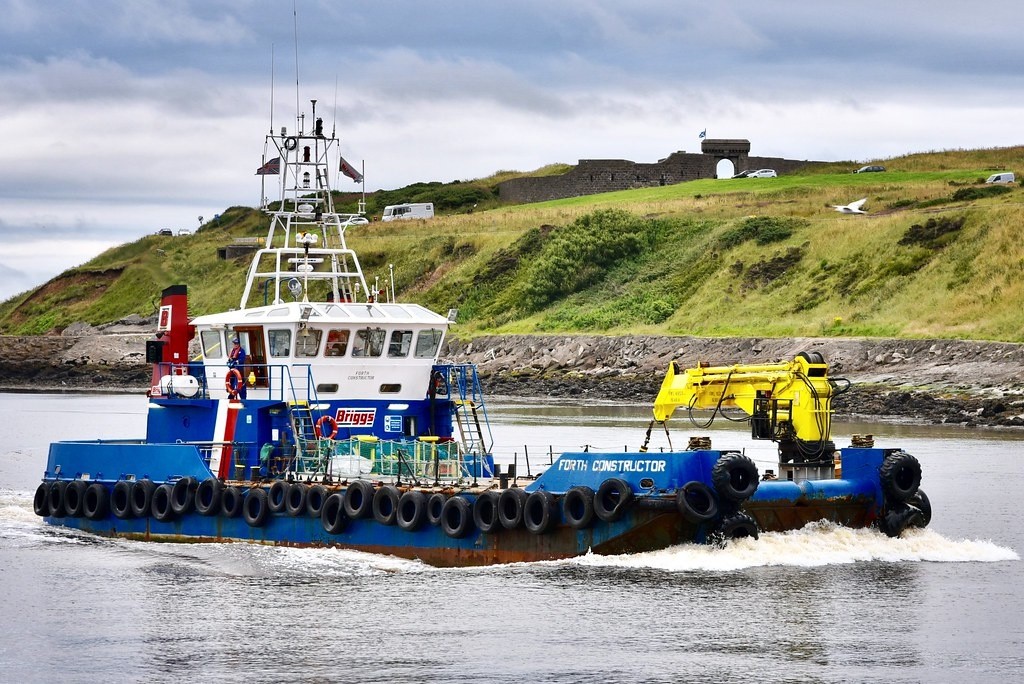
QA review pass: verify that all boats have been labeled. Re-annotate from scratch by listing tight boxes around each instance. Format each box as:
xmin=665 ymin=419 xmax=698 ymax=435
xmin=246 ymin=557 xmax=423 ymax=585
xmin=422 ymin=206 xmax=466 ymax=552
xmin=33 ymin=2 xmax=931 ymax=566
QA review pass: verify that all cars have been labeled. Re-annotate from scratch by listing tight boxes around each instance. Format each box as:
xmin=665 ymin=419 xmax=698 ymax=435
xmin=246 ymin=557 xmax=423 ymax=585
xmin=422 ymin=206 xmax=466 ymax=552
xmin=177 ymin=229 xmax=191 ymax=236
xmin=855 ymin=165 xmax=887 ymax=174
xmin=731 ymin=168 xmax=778 ymax=179
xmin=158 ymin=228 xmax=173 ymax=235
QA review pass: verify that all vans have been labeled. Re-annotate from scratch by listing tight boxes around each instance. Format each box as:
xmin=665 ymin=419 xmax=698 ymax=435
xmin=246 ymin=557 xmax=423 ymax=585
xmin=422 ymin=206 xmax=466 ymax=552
xmin=985 ymin=173 xmax=1015 ymax=185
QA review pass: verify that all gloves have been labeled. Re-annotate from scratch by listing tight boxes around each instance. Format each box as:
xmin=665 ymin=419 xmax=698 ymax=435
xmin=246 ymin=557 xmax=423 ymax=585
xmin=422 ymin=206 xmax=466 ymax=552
xmin=228 ymin=362 xmax=231 ymax=368
xmin=230 ymin=361 xmax=237 ymax=368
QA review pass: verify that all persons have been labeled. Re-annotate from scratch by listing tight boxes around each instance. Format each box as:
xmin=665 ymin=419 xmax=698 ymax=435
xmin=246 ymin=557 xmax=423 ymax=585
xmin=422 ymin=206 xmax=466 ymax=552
xmin=226 ymin=339 xmax=246 ymax=400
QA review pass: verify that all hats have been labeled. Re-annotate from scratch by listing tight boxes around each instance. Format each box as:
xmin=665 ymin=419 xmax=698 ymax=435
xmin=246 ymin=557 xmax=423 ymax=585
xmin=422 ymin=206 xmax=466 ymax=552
xmin=232 ymin=339 xmax=240 ymax=343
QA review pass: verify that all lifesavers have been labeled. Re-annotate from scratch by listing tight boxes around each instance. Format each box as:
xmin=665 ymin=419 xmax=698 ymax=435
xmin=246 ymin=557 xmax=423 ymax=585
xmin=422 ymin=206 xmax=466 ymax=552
xmin=676 ymin=480 xmax=719 ymax=524
xmin=882 ymin=488 xmax=932 ymax=539
xmin=343 ymin=480 xmax=473 ymax=538
xmin=33 ymin=480 xmax=110 ymax=521
xmin=713 ymin=510 xmax=760 ymax=550
xmin=225 ymin=367 xmax=243 ymax=396
xmin=472 ymin=487 xmax=560 ymax=536
xmin=110 ymin=476 xmax=268 ymax=527
xmin=563 ymin=476 xmax=635 ymax=531
xmin=711 ymin=451 xmax=760 ymax=503
xmin=267 ymin=480 xmax=350 ymax=535
xmin=315 ymin=415 xmax=338 ymax=439
xmin=879 ymin=450 xmax=923 ymax=502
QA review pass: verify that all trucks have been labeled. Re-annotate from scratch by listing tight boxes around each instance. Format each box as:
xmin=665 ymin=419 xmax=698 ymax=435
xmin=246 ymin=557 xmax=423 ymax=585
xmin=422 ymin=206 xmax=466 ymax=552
xmin=382 ymin=202 xmax=434 ymax=223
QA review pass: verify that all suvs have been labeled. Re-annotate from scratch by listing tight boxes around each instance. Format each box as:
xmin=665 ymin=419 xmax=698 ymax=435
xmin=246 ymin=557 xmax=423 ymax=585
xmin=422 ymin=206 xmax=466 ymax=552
xmin=342 ymin=217 xmax=369 ymax=226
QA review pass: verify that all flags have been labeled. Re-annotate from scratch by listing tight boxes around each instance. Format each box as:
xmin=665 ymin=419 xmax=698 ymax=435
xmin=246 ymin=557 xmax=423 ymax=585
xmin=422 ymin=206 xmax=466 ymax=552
xmin=699 ymin=131 xmax=705 ymax=138
xmin=339 ymin=156 xmax=363 ymax=183
xmin=256 ymin=156 xmax=280 ymax=175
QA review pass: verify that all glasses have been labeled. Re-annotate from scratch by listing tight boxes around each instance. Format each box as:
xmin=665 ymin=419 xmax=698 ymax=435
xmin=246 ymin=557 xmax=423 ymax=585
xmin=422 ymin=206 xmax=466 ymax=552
xmin=234 ymin=342 xmax=239 ymax=345
